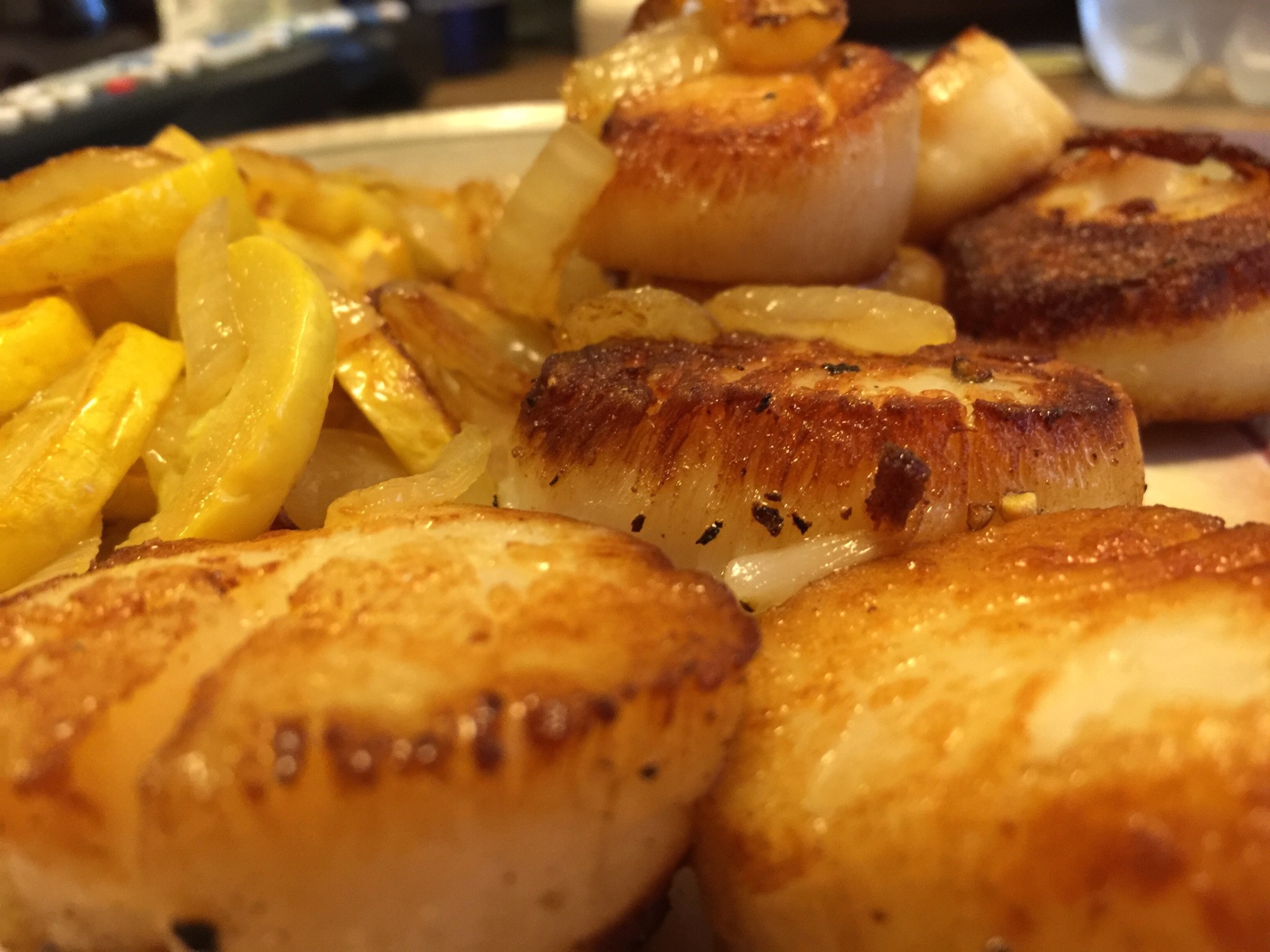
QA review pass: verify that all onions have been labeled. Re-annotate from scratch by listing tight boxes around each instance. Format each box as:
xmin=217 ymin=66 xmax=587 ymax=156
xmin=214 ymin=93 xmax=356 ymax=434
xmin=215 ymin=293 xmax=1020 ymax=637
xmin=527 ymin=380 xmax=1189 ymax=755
xmin=313 ymin=20 xmax=955 ymax=602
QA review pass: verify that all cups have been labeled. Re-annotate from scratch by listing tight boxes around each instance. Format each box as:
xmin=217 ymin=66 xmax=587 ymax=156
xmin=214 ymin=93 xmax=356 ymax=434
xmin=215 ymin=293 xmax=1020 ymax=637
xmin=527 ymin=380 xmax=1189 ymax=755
xmin=1225 ymin=0 xmax=1270 ymax=108
xmin=1079 ymin=0 xmax=1239 ymax=103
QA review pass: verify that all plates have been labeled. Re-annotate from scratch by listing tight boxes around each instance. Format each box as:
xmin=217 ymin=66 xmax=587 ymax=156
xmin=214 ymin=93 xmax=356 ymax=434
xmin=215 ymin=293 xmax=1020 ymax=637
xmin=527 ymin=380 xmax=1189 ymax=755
xmin=228 ymin=99 xmax=1269 ymax=531
